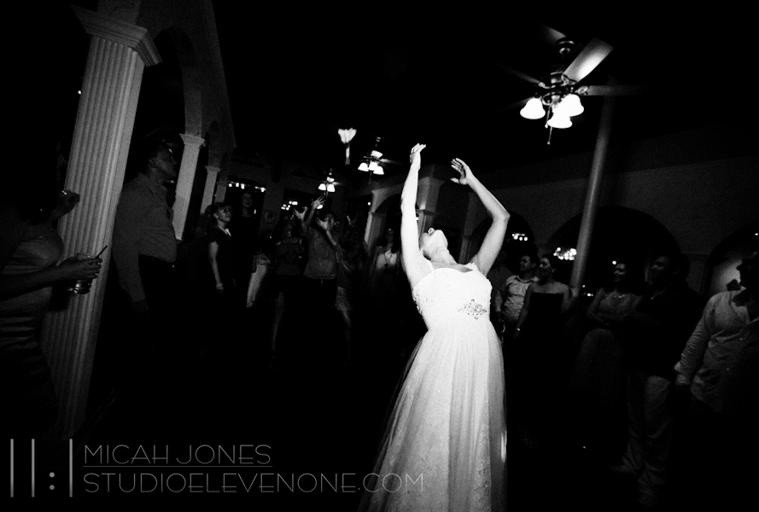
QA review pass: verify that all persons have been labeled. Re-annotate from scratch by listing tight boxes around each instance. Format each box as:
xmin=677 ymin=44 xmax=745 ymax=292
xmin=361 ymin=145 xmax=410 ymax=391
xmin=0 ymin=176 xmax=101 ymax=449
xmin=82 ymin=138 xmax=183 ymax=442
xmin=360 ymin=141 xmax=513 ymax=511
xmin=196 ymin=188 xmax=401 ymax=361
xmin=489 ymin=241 xmax=759 ymax=511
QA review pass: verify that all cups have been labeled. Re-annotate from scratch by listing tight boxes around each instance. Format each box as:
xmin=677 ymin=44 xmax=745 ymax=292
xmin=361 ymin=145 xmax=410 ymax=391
xmin=73 ymin=252 xmax=93 ymax=294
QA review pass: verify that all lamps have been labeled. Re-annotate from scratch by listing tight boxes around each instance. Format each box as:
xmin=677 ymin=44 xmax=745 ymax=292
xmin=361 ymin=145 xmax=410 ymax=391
xmin=318 ymin=176 xmax=337 ymax=195
xmin=524 ymin=92 xmax=584 ymax=131
xmin=357 ymin=161 xmax=387 ymax=176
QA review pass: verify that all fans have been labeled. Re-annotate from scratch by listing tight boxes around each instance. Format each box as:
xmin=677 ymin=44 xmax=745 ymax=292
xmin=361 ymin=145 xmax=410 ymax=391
xmin=495 ymin=34 xmax=624 ymax=135
xmin=353 ymin=150 xmax=396 ymax=180
